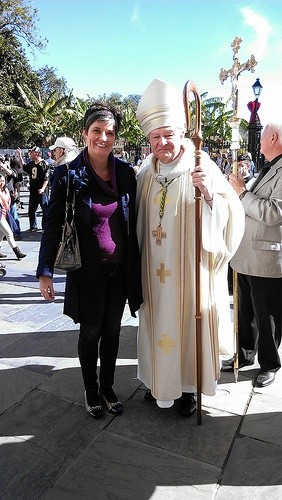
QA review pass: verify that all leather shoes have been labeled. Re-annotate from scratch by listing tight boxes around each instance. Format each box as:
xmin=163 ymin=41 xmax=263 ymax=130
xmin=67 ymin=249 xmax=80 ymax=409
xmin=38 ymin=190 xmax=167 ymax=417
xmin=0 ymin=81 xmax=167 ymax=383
xmin=221 ymin=360 xmax=246 ymax=372
xmin=254 ymin=369 xmax=275 ymax=387
xmin=145 ymin=388 xmax=157 ymax=403
xmin=177 ymin=400 xmax=197 ymax=418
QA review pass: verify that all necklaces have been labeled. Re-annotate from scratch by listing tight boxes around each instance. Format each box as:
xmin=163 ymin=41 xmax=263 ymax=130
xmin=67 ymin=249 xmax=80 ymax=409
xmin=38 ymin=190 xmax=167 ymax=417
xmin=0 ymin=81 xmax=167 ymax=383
xmin=152 ymin=187 xmax=167 ymax=245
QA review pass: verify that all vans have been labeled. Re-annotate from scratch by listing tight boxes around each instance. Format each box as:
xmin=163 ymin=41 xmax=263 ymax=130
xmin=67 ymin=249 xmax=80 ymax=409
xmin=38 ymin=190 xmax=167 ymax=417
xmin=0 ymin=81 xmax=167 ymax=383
xmin=112 ymin=145 xmax=125 ymax=159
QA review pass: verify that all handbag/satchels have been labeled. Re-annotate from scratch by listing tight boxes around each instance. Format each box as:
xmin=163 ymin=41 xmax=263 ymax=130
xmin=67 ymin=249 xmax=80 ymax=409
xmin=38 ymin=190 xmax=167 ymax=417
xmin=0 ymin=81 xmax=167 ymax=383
xmin=6 ymin=209 xmax=19 ymax=233
xmin=54 ymin=162 xmax=82 ymax=272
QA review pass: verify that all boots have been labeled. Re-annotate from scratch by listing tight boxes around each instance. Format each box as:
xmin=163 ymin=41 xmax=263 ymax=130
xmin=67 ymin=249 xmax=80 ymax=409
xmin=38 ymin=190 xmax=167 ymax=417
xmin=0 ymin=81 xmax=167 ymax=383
xmin=0 ymin=246 xmax=7 ymax=258
xmin=13 ymin=246 xmax=26 ymax=260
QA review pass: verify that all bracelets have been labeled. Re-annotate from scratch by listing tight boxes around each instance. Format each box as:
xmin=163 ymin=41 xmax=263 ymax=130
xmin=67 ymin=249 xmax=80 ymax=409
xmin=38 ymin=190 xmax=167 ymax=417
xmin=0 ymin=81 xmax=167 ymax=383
xmin=204 ymin=196 xmax=213 ymax=201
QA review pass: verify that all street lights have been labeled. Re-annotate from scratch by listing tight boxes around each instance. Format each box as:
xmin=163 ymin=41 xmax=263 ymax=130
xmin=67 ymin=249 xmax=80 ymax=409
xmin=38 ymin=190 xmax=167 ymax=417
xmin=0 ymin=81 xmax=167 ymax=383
xmin=247 ymin=78 xmax=264 ymax=172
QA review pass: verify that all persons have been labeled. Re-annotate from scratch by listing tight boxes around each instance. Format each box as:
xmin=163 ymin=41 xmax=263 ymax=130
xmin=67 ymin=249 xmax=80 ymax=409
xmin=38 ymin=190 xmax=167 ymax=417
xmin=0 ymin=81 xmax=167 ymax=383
xmin=0 ymin=152 xmax=27 ymax=260
xmin=49 ymin=137 xmax=80 ymax=187
xmin=22 ymin=149 xmax=56 ymax=166
xmin=16 ymin=146 xmax=50 ymax=233
xmin=119 ymin=148 xmax=256 ymax=183
xmin=137 ymin=79 xmax=246 ymax=414
xmin=36 ymin=102 xmax=143 ymax=418
xmin=222 ymin=120 xmax=282 ymax=387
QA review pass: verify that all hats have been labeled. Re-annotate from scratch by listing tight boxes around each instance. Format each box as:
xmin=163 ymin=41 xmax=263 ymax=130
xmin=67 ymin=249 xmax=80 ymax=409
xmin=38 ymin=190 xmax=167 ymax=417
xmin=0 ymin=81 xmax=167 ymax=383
xmin=135 ymin=78 xmax=184 ymax=137
xmin=49 ymin=137 xmax=76 ymax=151
xmin=28 ymin=146 xmax=40 ymax=153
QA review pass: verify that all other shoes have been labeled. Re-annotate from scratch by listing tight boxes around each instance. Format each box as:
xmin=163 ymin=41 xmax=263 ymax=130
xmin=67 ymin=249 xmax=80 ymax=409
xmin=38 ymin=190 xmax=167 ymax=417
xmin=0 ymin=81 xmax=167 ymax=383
xmin=100 ymin=387 xmax=123 ymax=416
xmin=14 ymin=232 xmax=21 ymax=241
xmin=26 ymin=227 xmax=38 ymax=233
xmin=83 ymin=390 xmax=104 ymax=419
